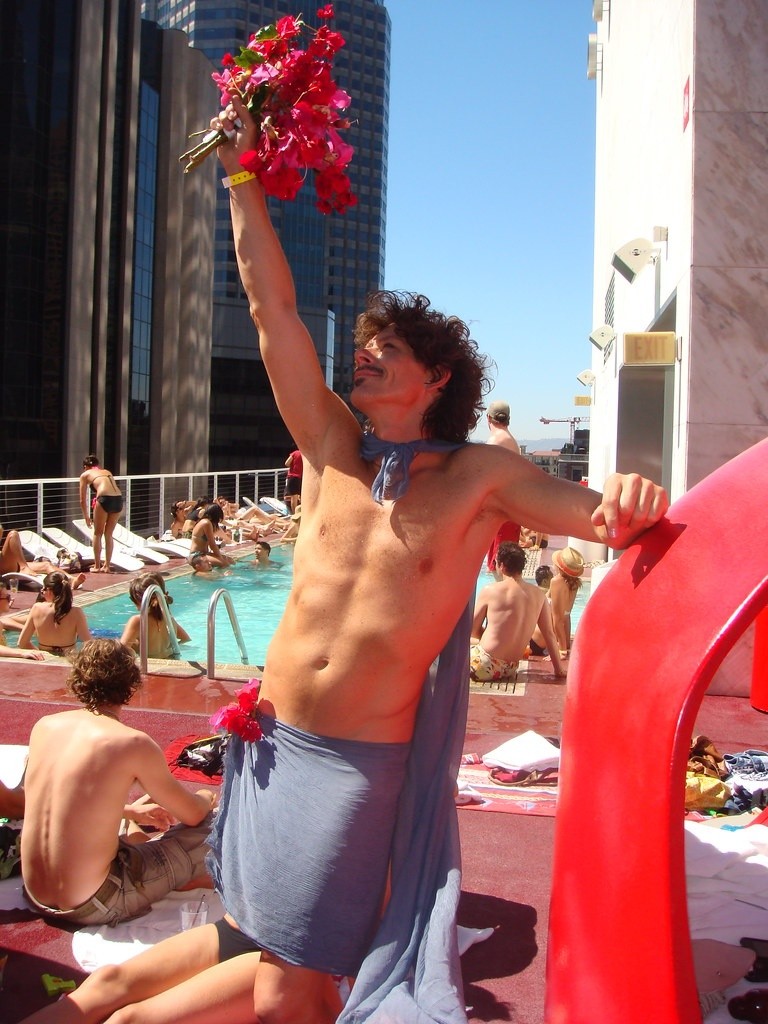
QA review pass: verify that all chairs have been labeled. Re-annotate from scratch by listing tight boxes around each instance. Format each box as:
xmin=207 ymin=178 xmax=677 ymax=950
xmin=243 ymin=496 xmax=270 ymax=517
xmin=17 ymin=530 xmax=62 ymax=567
xmin=160 ymin=530 xmax=193 ymax=550
xmin=72 ymin=518 xmax=170 ymax=564
xmin=112 ymin=522 xmax=192 ymax=559
xmin=42 ymin=527 xmax=145 ymax=572
xmin=260 ymin=497 xmax=289 ymax=516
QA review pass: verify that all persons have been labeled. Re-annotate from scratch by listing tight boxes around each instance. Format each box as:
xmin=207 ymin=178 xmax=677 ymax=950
xmin=118 ymin=578 xmax=177 ymax=660
xmin=0 ymin=761 xmax=30 ymax=883
xmin=17 ymin=571 xmax=94 ymax=656
xmin=0 ymin=582 xmax=46 ymax=660
xmin=78 ymin=455 xmax=122 ymax=574
xmin=19 ymin=637 xmax=219 ymax=926
xmin=168 ymin=443 xmax=302 ymax=577
xmin=466 ymin=403 xmax=582 ymax=679
xmin=138 ymin=571 xmax=173 ymax=613
xmin=205 ymin=104 xmax=670 ymax=1024
xmin=0 ymin=525 xmax=85 ymax=590
xmin=15 ymin=863 xmax=399 ymax=1024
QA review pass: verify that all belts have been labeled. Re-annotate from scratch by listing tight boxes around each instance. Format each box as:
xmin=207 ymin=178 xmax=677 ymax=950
xmin=22 ymin=859 xmax=122 ymax=921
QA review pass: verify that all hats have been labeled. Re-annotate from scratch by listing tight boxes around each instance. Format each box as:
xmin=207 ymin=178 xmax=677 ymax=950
xmin=489 ymin=400 xmax=510 ymax=421
xmin=551 ymin=547 xmax=585 ymax=578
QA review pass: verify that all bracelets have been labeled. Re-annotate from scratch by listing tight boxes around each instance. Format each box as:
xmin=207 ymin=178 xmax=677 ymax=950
xmin=221 ymin=170 xmax=256 ymax=188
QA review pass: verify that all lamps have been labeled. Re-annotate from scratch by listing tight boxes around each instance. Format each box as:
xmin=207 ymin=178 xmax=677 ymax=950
xmin=611 ymin=237 xmax=660 ymax=283
xmin=588 ymin=324 xmax=617 ymax=351
xmin=577 ymin=369 xmax=597 ymax=387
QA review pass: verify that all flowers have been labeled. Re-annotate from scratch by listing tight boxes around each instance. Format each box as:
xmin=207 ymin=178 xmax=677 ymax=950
xmin=210 ymin=677 xmax=264 ymax=745
xmin=177 ymin=4 xmax=363 ymax=219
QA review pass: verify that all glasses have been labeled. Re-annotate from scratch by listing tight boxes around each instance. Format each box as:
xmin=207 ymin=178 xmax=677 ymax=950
xmin=41 ymin=587 xmax=48 ymax=595
xmin=0 ymin=595 xmax=11 ymax=602
